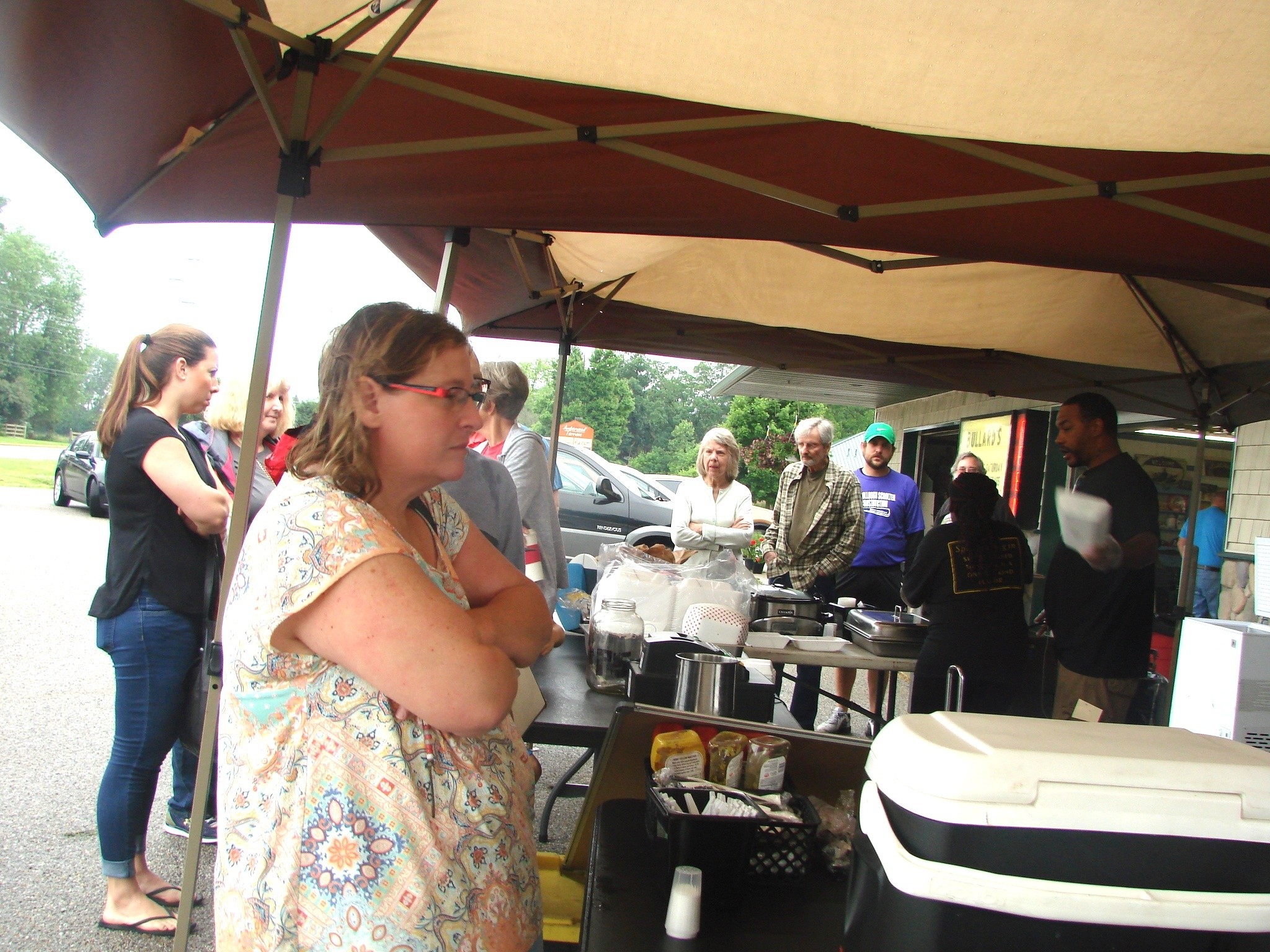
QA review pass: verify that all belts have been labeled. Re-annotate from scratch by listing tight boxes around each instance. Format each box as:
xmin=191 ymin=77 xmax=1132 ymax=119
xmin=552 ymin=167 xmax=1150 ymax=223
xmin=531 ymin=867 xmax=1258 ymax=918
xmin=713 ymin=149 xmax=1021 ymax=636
xmin=1198 ymin=566 xmax=1220 ymax=572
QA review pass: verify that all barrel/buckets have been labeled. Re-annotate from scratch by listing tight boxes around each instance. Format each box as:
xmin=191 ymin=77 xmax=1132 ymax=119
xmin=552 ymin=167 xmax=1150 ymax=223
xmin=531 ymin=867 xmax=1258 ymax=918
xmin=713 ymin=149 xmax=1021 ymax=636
xmin=673 ymin=652 xmax=741 ymax=718
xmin=749 ymin=616 xmax=824 ymax=636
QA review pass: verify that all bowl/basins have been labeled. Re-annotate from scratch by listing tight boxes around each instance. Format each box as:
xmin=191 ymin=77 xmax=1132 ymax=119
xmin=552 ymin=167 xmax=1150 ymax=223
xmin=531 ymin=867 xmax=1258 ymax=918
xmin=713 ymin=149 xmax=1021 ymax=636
xmin=786 ymin=635 xmax=852 ymax=652
xmin=745 ymin=632 xmax=791 ymax=649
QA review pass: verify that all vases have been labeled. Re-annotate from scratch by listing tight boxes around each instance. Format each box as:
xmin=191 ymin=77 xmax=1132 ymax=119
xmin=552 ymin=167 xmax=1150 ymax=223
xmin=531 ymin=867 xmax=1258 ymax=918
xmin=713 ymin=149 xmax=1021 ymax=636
xmin=743 ymin=556 xmax=765 ymax=574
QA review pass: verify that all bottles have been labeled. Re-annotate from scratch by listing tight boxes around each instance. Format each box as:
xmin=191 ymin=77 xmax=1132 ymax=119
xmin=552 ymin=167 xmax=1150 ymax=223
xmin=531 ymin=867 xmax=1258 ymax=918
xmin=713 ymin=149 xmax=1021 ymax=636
xmin=584 ymin=598 xmax=645 ymax=696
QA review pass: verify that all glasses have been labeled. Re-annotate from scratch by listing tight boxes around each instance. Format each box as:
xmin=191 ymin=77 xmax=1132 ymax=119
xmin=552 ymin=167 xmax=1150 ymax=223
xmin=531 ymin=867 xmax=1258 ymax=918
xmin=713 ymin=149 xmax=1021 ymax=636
xmin=383 ymin=382 xmax=487 ymax=411
xmin=474 ymin=377 xmax=492 ymax=391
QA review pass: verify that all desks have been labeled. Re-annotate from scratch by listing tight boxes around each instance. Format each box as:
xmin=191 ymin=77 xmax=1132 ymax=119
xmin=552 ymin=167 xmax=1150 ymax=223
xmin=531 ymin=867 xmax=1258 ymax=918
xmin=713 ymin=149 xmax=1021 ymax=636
xmin=521 ymin=628 xmax=805 ymax=845
xmin=737 ymin=631 xmax=920 ymax=736
xmin=581 ymin=796 xmax=843 ymax=952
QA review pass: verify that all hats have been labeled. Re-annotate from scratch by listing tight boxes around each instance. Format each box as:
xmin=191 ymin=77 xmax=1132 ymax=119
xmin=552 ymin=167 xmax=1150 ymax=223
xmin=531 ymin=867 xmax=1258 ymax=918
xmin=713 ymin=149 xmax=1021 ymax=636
xmin=863 ymin=422 xmax=896 ymax=445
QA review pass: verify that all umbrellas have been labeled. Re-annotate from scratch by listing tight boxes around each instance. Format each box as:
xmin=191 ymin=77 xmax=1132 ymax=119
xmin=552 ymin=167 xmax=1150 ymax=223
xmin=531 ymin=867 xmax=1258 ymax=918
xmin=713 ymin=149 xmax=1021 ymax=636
xmin=0 ymin=0 xmax=1270 ymax=952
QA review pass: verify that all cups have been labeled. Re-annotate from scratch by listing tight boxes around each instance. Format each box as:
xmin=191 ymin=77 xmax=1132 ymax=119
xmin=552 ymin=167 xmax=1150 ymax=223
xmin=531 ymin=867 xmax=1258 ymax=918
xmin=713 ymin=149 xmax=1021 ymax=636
xmin=664 ymin=866 xmax=702 ymax=940
xmin=826 ymin=623 xmax=838 ymax=637
xmin=822 ymin=624 xmax=834 ymax=637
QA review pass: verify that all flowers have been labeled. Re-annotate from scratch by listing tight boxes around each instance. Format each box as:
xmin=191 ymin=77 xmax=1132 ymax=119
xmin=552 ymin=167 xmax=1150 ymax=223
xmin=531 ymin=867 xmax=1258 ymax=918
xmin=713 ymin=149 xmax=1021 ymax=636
xmin=741 ymin=532 xmax=767 ymax=564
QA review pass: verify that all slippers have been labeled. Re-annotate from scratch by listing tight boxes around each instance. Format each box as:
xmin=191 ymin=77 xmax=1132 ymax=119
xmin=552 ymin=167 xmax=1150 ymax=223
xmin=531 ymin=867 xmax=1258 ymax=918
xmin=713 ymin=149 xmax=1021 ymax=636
xmin=99 ymin=908 xmax=197 ymax=936
xmin=147 ymin=883 xmax=203 ymax=905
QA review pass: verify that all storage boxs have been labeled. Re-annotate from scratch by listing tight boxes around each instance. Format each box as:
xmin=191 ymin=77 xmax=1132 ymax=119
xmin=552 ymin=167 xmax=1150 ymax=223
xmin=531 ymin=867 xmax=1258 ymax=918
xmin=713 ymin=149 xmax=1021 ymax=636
xmin=843 ymin=710 xmax=1270 ymax=952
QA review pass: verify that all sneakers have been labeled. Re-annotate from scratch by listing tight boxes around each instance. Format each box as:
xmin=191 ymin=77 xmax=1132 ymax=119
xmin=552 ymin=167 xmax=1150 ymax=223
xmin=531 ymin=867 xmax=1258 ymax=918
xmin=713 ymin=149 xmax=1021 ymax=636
xmin=869 ymin=718 xmax=883 ymax=738
xmin=817 ymin=707 xmax=851 ymax=732
xmin=164 ymin=806 xmax=218 ymax=843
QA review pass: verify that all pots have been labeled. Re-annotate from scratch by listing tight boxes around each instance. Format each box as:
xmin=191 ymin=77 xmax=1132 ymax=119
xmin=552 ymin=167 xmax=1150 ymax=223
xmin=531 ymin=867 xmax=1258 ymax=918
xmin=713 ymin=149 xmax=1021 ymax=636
xmin=749 ymin=583 xmax=823 ymax=621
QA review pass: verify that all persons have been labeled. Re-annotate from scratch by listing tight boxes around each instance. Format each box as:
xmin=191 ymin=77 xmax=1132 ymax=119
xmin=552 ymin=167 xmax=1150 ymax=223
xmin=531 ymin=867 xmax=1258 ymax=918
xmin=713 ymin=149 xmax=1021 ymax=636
xmin=527 ymin=620 xmax=565 ymax=785
xmin=759 ymin=416 xmax=866 ymax=731
xmin=816 ymin=423 xmax=926 ymax=737
xmin=1031 ymin=392 xmax=1164 ymax=724
xmin=1176 ymin=487 xmax=1227 ymax=619
xmin=213 ymin=301 xmax=553 ymax=952
xmin=671 ymin=427 xmax=754 ymax=566
xmin=900 ymin=472 xmax=1034 ymax=715
xmin=439 ymin=447 xmax=525 ymax=577
xmin=89 ymin=323 xmax=231 ymax=935
xmin=465 ymin=361 xmax=569 ymax=617
xmin=934 ymin=452 xmax=1017 ymax=527
xmin=166 ymin=353 xmax=296 ymax=842
xmin=518 ymin=423 xmax=563 ymax=514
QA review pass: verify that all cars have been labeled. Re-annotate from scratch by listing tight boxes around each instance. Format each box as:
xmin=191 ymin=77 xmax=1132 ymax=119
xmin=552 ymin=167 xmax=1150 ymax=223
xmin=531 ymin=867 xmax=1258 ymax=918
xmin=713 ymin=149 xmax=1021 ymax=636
xmin=541 ymin=438 xmax=681 ymax=568
xmin=53 ymin=430 xmax=113 ymax=518
xmin=608 ymin=464 xmax=775 ymax=541
